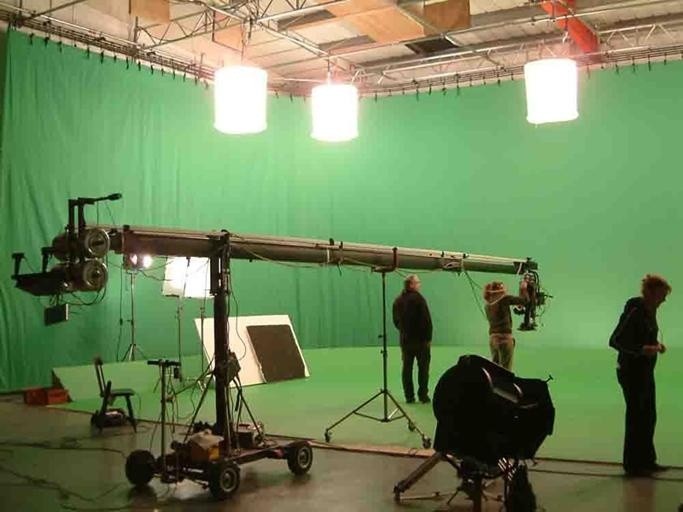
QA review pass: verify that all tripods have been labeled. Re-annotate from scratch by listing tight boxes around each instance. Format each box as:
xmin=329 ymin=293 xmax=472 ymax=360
xmin=324 ymin=273 xmax=431 ymax=448
xmin=122 ymin=274 xmax=148 ymax=362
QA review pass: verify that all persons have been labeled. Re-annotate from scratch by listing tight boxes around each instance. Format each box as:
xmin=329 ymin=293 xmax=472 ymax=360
xmin=483 ymin=279 xmax=529 ymax=374
xmin=609 ymin=276 xmax=672 ymax=476
xmin=392 ymin=275 xmax=433 ymax=404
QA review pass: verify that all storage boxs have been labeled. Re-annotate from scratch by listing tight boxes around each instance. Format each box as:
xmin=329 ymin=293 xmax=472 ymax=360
xmin=24 ymin=389 xmax=68 ymax=405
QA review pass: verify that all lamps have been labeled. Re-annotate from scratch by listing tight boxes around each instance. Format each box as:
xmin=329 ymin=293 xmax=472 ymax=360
xmin=311 ymin=57 xmax=358 ymax=142
xmin=523 ymin=15 xmax=579 ymax=124
xmin=213 ymin=20 xmax=267 ymax=135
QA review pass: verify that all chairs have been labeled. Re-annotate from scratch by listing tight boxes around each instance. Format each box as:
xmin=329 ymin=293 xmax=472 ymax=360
xmin=94 ymin=355 xmax=138 ymax=434
